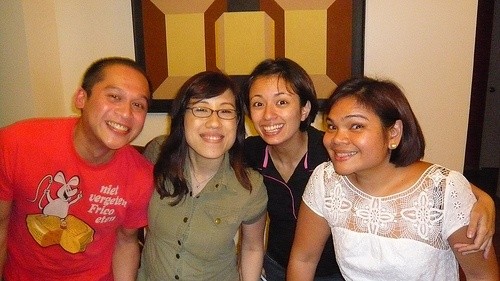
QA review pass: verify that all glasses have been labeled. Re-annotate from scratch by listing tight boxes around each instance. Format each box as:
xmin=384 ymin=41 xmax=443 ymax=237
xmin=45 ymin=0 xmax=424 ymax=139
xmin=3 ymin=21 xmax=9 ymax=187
xmin=185 ymin=105 xmax=241 ymax=121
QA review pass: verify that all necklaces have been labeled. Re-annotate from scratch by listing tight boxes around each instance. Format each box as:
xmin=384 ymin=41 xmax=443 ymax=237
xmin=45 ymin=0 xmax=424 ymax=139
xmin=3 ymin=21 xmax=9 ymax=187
xmin=189 ymin=165 xmax=215 ymax=190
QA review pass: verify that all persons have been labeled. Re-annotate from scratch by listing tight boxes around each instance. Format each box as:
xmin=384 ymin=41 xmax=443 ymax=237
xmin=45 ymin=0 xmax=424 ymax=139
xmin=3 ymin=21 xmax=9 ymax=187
xmin=0 ymin=56 xmax=156 ymax=281
xmin=237 ymin=57 xmax=495 ymax=281
xmin=133 ymin=70 xmax=269 ymax=281
xmin=287 ymin=77 xmax=500 ymax=281
